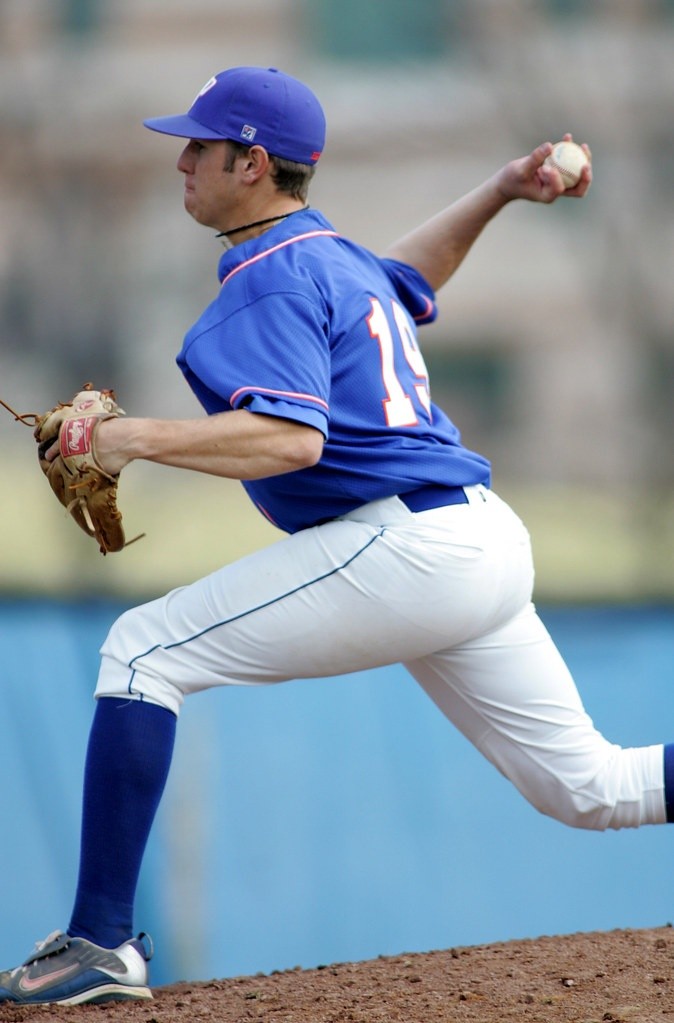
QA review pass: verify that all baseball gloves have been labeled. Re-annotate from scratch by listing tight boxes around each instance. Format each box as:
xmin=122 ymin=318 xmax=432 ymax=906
xmin=33 ymin=380 xmax=129 ymax=557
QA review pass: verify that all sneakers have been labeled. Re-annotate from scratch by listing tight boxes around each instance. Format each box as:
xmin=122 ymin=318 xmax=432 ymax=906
xmin=0 ymin=930 xmax=153 ymax=1006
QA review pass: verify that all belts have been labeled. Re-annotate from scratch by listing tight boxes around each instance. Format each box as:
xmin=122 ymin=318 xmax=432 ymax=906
xmin=397 ymin=485 xmax=468 ymax=514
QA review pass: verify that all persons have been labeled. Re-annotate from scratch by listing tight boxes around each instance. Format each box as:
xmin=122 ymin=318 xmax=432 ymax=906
xmin=0 ymin=65 xmax=674 ymax=1006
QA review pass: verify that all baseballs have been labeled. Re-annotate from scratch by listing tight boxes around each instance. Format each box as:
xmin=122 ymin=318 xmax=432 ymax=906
xmin=534 ymin=138 xmax=589 ymax=192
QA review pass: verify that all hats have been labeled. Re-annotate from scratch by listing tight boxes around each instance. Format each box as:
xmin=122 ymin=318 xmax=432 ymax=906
xmin=143 ymin=67 xmax=326 ymax=165
